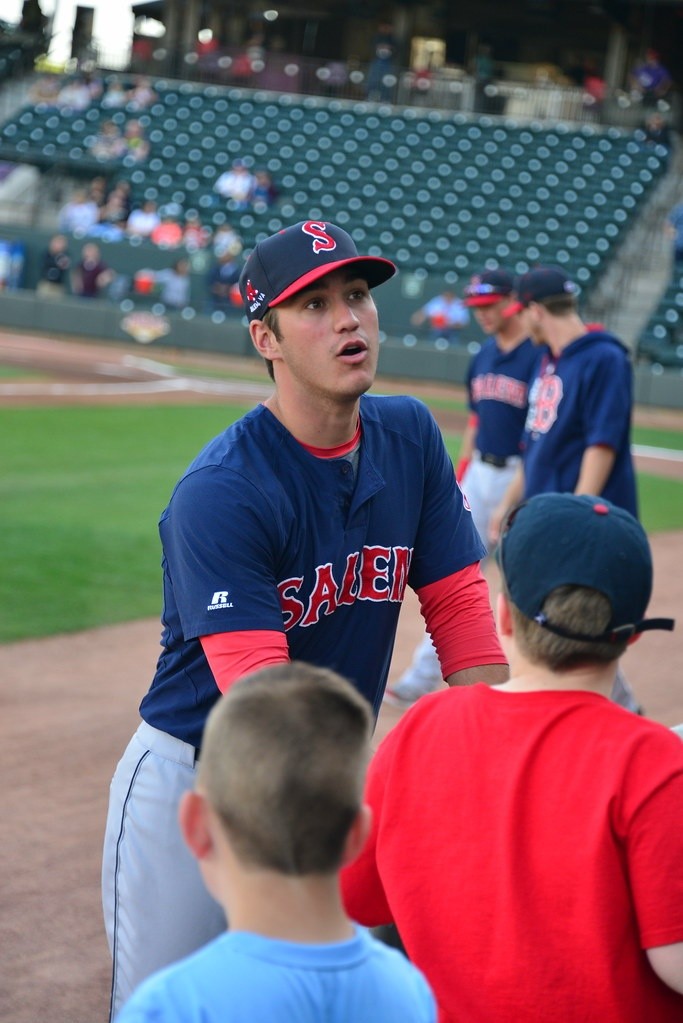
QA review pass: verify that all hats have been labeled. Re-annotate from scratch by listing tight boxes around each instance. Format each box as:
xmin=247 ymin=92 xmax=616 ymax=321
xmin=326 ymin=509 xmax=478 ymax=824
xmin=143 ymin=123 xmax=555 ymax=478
xmin=500 ymin=268 xmax=575 ymax=317
xmin=495 ymin=492 xmax=674 ymax=641
xmin=463 ymin=268 xmax=513 ymax=305
xmin=237 ymin=220 xmax=396 ymax=323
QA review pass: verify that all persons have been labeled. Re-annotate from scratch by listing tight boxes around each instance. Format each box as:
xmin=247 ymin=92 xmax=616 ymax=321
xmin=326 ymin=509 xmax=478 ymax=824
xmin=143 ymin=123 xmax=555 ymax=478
xmin=0 ymin=176 xmax=249 ymax=319
xmin=132 ymin=26 xmax=674 ymax=148
xmin=338 ymin=493 xmax=683 ymax=1023
xmin=213 ymin=160 xmax=276 ymax=210
xmin=488 ymin=272 xmax=647 ymax=715
xmin=666 ymin=200 xmax=683 ymax=263
xmin=113 ymin=661 xmax=438 ymax=1023
xmin=103 ymin=221 xmax=508 ymax=1023
xmin=30 ymin=70 xmax=157 ymax=113
xmin=383 ymin=269 xmax=548 ymax=706
xmin=90 ymin=120 xmax=150 ymax=164
xmin=409 ymin=285 xmax=469 ymax=345
